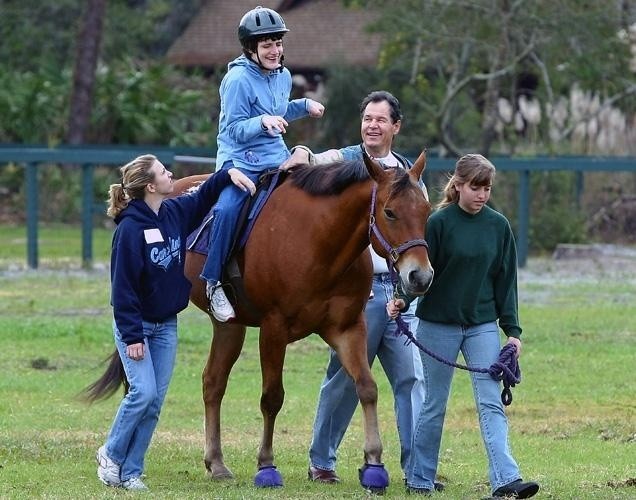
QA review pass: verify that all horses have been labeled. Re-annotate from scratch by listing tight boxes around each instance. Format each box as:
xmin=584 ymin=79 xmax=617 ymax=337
xmin=71 ymin=143 xmax=435 ymax=497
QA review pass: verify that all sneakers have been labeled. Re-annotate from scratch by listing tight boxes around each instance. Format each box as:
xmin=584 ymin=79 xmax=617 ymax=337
xmin=95 ymin=445 xmax=122 ymax=486
xmin=117 ymin=475 xmax=148 ymax=493
xmin=206 ymin=278 xmax=238 ymax=323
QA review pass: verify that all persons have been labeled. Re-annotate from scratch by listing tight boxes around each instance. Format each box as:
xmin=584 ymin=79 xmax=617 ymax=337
xmin=396 ymin=151 xmax=540 ymax=500
xmin=276 ymin=87 xmax=448 ymax=494
xmin=93 ymin=153 xmax=259 ymax=492
xmin=188 ymin=5 xmax=327 ymax=326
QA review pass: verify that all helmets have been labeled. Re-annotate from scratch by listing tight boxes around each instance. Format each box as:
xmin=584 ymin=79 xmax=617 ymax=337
xmin=237 ymin=4 xmax=290 ymax=42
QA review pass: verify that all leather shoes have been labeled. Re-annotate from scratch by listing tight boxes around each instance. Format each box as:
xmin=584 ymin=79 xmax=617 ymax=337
xmin=491 ymin=480 xmax=540 ymax=500
xmin=308 ymin=463 xmax=342 ymax=486
xmin=401 ymin=473 xmax=446 ymax=497
